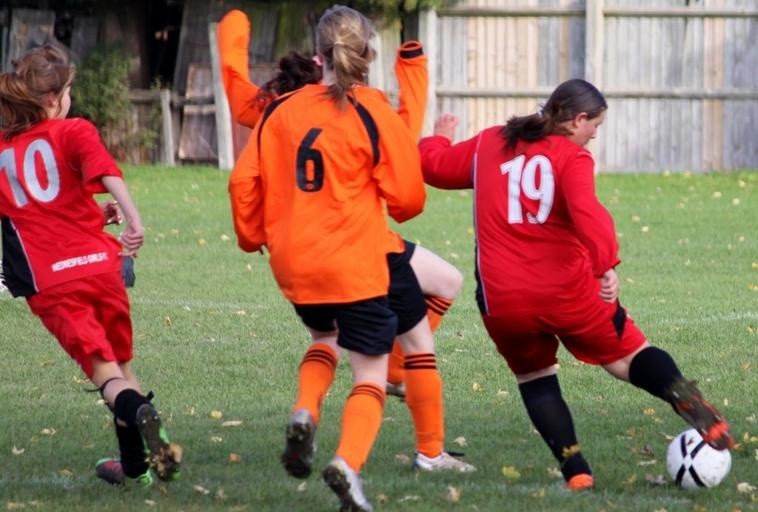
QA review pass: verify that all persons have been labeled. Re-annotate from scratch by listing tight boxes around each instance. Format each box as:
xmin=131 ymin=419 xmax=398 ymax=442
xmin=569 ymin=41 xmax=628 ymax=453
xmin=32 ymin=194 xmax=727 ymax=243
xmin=225 ymin=4 xmax=429 ymax=512
xmin=216 ymin=9 xmax=480 ymax=478
xmin=417 ymin=77 xmax=738 ymax=494
xmin=0 ymin=39 xmax=185 ymax=492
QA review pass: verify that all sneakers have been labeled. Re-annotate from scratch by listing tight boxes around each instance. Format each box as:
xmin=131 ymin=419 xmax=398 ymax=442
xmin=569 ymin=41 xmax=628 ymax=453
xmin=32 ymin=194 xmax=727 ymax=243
xmin=322 ymin=457 xmax=374 ymax=512
xmin=567 ymin=471 xmax=593 ymax=491
xmin=386 ymin=380 xmax=403 ymax=399
xmin=667 ymin=377 xmax=733 ymax=451
xmin=95 ymin=458 xmax=154 ymax=488
xmin=129 ymin=397 xmax=183 ymax=483
xmin=280 ymin=411 xmax=314 ymax=478
xmin=415 ymin=452 xmax=477 ymax=473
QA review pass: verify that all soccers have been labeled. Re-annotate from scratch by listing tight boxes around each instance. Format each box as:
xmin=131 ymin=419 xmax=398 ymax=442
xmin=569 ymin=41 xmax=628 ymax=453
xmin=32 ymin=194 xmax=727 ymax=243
xmin=667 ymin=428 xmax=731 ymax=490
